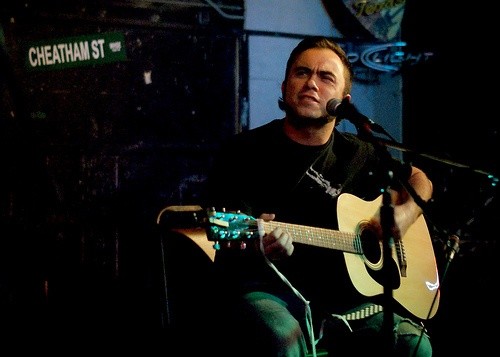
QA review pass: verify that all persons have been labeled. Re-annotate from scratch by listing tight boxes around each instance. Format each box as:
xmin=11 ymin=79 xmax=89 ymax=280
xmin=179 ymin=35 xmax=436 ymax=357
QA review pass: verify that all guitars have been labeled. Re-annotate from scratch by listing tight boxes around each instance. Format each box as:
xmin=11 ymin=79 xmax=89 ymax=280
xmin=156 ymin=182 xmax=440 ymax=320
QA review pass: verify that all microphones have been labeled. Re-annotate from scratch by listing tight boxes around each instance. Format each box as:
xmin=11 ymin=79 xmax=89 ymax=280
xmin=444 ymin=235 xmax=461 ymax=262
xmin=326 ymin=98 xmax=385 ymax=135
